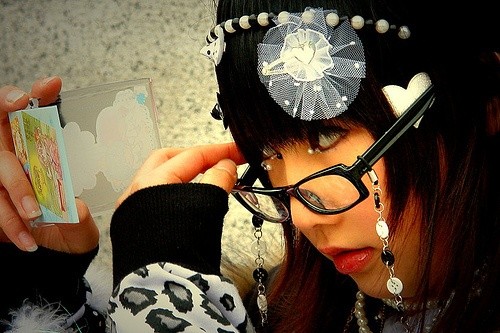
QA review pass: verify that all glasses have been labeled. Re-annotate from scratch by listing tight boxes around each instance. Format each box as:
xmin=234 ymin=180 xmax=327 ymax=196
xmin=229 ymin=81 xmax=449 ymax=223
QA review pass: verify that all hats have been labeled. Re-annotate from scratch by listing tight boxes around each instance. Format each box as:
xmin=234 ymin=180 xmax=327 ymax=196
xmin=211 ymin=0 xmax=500 ymax=137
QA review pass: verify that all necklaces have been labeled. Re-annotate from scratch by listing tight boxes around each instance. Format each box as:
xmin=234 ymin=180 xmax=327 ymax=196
xmin=353 ymin=290 xmax=374 ymax=333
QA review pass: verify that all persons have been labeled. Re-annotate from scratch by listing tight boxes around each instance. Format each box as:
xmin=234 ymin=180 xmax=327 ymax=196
xmin=0 ymin=0 xmax=500 ymax=333
